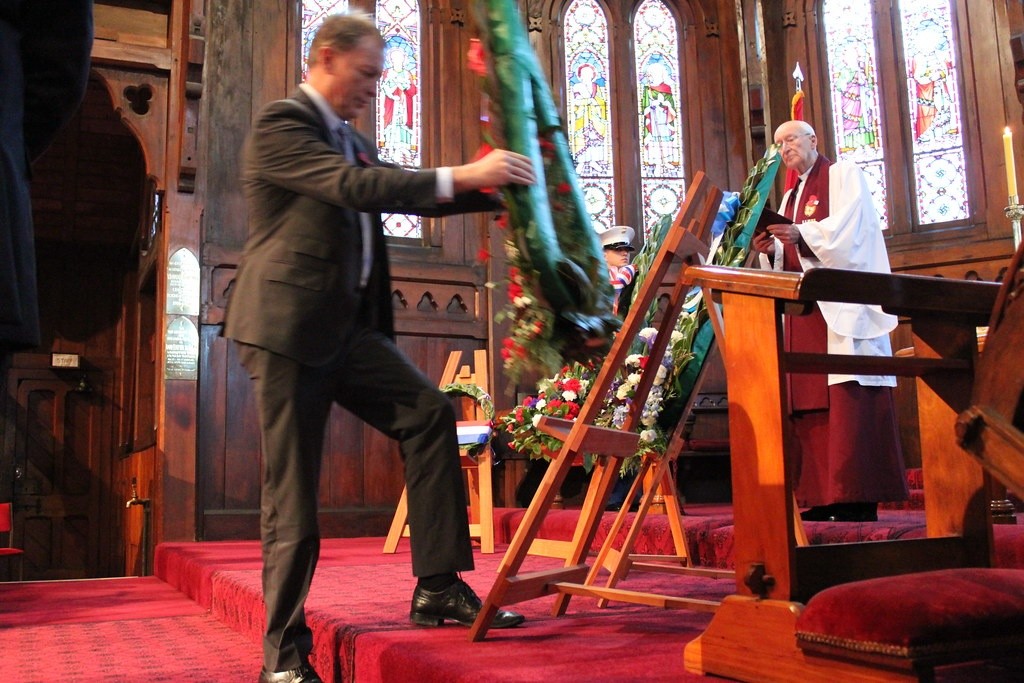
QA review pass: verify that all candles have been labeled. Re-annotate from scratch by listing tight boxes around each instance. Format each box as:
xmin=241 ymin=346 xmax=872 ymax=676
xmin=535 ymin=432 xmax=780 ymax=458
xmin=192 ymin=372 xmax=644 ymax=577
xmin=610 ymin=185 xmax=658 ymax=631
xmin=1002 ymin=127 xmax=1020 ymax=205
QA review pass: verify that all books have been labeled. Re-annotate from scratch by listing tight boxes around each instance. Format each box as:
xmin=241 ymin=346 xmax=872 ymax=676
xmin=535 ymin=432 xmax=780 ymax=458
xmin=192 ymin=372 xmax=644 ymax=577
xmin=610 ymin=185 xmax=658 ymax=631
xmin=754 ymin=207 xmax=795 ymax=238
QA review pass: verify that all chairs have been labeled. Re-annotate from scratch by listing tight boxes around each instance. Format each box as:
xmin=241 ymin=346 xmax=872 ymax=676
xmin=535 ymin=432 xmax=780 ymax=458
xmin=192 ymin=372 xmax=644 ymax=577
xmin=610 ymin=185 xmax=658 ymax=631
xmin=0 ymin=503 xmax=24 ymax=581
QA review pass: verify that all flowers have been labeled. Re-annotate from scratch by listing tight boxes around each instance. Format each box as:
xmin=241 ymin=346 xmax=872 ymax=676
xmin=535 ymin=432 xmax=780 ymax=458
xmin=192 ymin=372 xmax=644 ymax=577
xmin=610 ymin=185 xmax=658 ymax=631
xmin=489 ymin=325 xmax=685 ymax=464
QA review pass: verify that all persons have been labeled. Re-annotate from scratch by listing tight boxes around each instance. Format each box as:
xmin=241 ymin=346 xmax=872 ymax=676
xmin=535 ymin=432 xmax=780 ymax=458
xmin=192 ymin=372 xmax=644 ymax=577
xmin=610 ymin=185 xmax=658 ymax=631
xmin=217 ymin=15 xmax=537 ymax=683
xmin=597 ymin=226 xmax=640 ymax=325
xmin=0 ymin=0 xmax=96 ymax=430
xmin=749 ymin=119 xmax=909 ymax=523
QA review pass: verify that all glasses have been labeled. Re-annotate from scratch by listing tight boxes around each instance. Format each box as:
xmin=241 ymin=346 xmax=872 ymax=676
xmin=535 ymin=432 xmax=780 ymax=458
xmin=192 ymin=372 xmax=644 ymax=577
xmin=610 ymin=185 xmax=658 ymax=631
xmin=775 ymin=133 xmax=810 ymax=149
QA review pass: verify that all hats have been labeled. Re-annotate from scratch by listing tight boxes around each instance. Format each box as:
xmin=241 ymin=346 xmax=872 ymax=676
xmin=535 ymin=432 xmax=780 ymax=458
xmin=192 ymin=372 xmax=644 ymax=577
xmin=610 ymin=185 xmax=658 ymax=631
xmin=599 ymin=226 xmax=636 ymax=251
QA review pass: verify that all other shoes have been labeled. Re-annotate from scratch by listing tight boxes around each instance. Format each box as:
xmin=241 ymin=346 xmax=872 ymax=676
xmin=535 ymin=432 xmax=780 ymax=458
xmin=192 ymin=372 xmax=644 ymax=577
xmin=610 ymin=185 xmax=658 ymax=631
xmin=801 ymin=500 xmax=879 ymax=522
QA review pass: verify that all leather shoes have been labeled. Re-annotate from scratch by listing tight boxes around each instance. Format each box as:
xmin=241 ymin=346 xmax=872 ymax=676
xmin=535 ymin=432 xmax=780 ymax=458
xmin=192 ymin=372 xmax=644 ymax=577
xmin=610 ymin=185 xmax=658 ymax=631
xmin=258 ymin=659 xmax=324 ymax=683
xmin=409 ymin=579 xmax=526 ymax=628
